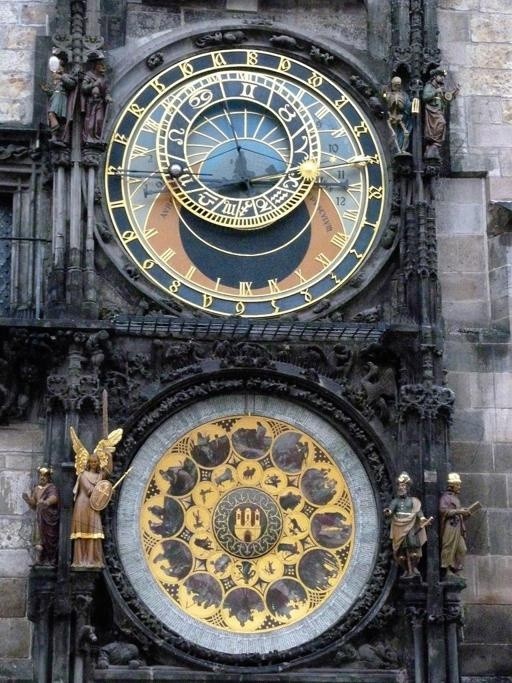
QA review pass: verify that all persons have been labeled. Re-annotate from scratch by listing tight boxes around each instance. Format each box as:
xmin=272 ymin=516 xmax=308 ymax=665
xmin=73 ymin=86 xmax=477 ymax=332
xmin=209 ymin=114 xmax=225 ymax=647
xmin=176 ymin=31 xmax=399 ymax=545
xmin=23 ymin=465 xmax=61 ymax=565
xmin=422 ymin=65 xmax=459 ymax=158
xmin=439 ymin=471 xmax=471 ymax=583
xmin=381 ymin=470 xmax=428 ymax=584
xmin=40 ymin=54 xmax=76 ymax=138
xmin=70 ymin=452 xmax=107 ymax=566
xmin=82 ymin=48 xmax=111 ymax=140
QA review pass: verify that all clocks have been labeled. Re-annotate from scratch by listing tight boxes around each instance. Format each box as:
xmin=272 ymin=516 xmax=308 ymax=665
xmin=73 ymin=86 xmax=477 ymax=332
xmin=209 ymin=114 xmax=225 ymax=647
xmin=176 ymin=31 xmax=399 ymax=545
xmin=105 ymin=47 xmax=402 ymax=336
